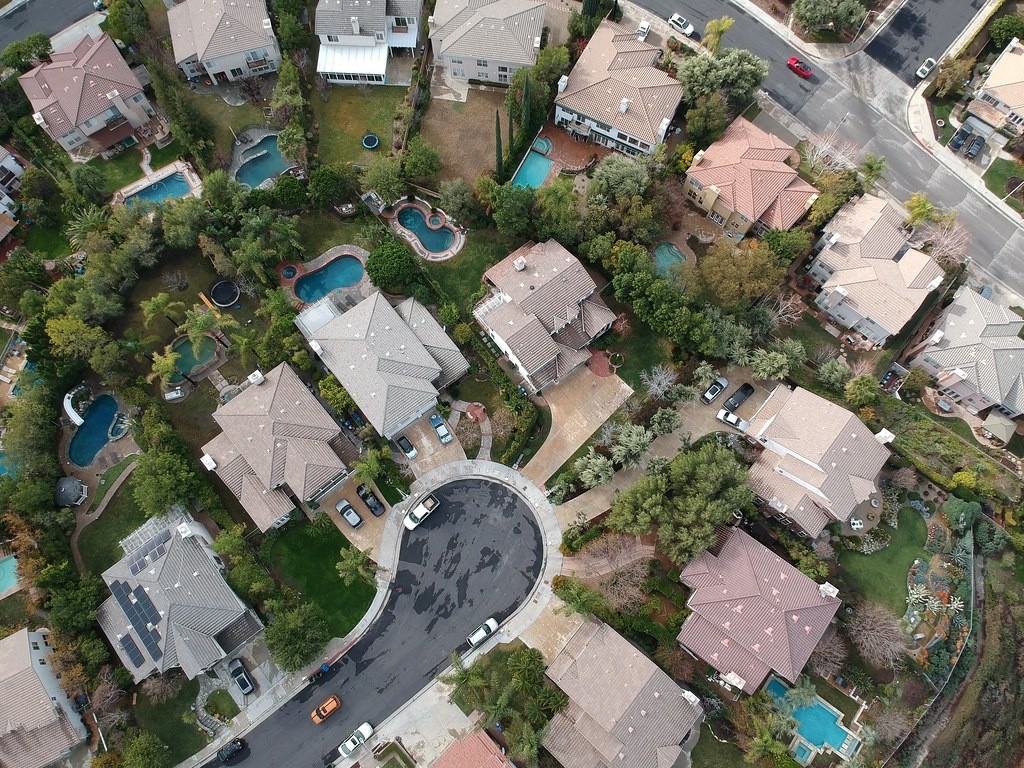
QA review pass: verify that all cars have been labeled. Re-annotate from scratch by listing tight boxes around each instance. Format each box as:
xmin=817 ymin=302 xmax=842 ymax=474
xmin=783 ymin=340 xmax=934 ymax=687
xmin=338 ymin=722 xmax=374 ymax=758
xmin=667 ymin=12 xmax=694 ymax=37
xmin=356 ymin=482 xmax=386 ymax=517
xmin=723 ymin=383 xmax=755 ymax=414
xmin=396 ymin=435 xmax=419 ymax=460
xmin=466 ymin=618 xmax=499 ymax=649
xmin=699 ymin=376 xmax=728 ymax=405
xmin=429 ymin=413 xmax=453 ymax=444
xmin=311 ymin=694 xmax=342 ymax=724
xmin=228 ymin=658 xmax=255 ymax=695
xmin=335 ymin=498 xmax=365 ymax=530
xmin=217 ymin=738 xmax=243 ymax=763
xmin=636 ymin=20 xmax=651 ymax=42
xmin=916 ymin=57 xmax=937 ymax=79
xmin=786 ymin=57 xmax=812 ymax=79
xmin=715 ymin=408 xmax=750 ymax=433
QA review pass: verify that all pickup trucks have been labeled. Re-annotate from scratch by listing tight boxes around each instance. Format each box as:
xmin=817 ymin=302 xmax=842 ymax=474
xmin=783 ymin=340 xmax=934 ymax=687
xmin=402 ymin=493 xmax=440 ymax=531
